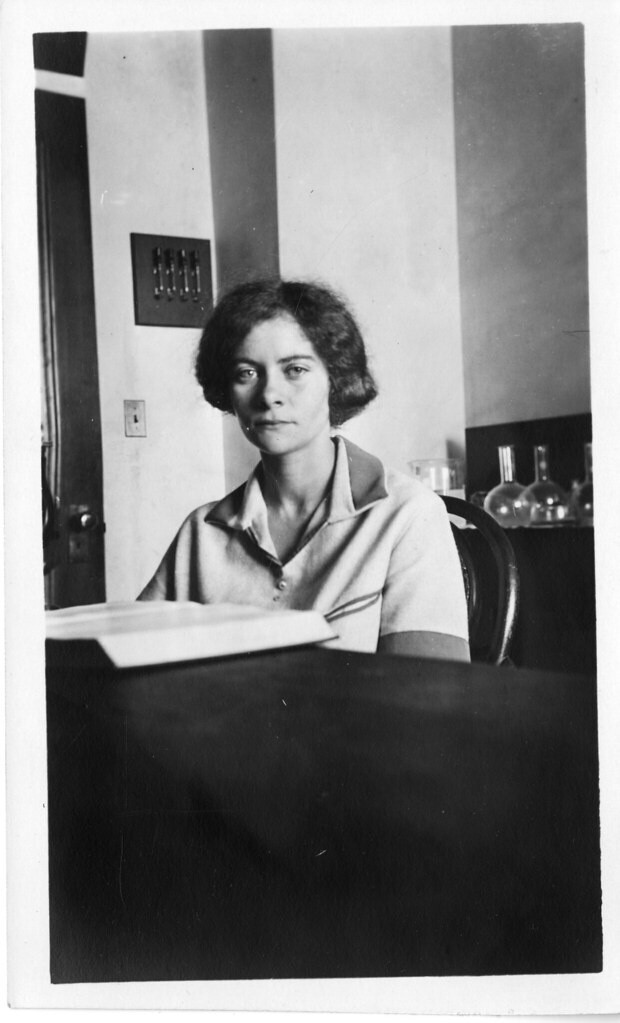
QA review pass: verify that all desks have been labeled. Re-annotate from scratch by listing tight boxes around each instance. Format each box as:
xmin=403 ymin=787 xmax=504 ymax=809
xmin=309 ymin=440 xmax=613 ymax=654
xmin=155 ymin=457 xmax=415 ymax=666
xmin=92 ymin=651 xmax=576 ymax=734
xmin=46 ymin=640 xmax=603 ymax=985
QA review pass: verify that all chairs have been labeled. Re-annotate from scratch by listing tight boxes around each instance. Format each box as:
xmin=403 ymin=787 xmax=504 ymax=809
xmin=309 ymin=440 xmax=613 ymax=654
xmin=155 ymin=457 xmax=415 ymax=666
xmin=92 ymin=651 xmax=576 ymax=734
xmin=441 ymin=496 xmax=520 ymax=668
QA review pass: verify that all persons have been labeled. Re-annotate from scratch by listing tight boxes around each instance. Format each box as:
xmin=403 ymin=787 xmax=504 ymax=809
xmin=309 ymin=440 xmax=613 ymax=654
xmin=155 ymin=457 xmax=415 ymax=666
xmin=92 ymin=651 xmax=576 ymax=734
xmin=132 ymin=279 xmax=471 ymax=662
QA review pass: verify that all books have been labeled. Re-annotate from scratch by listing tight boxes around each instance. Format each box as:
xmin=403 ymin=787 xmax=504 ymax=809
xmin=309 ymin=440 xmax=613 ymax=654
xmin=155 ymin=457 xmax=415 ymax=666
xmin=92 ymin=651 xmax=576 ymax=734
xmin=44 ymin=600 xmax=338 ymax=675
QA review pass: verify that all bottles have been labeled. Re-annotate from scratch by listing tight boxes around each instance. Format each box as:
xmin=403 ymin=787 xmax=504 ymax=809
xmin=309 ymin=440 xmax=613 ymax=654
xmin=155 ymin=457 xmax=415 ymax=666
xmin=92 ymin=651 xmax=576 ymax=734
xmin=483 ymin=443 xmax=533 ymax=527
xmin=514 ymin=444 xmax=569 ymax=527
xmin=571 ymin=441 xmax=594 ymax=527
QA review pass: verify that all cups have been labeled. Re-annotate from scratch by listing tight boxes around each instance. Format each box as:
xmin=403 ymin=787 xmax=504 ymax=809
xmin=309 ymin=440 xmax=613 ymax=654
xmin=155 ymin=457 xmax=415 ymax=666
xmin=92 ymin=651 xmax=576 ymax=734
xmin=409 ymin=458 xmax=465 ymax=500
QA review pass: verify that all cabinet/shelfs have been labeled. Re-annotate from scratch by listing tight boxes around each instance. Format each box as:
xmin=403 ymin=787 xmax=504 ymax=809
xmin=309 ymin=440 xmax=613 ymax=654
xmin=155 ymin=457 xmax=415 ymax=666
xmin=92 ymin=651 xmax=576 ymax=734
xmin=458 ymin=413 xmax=597 ymax=673
